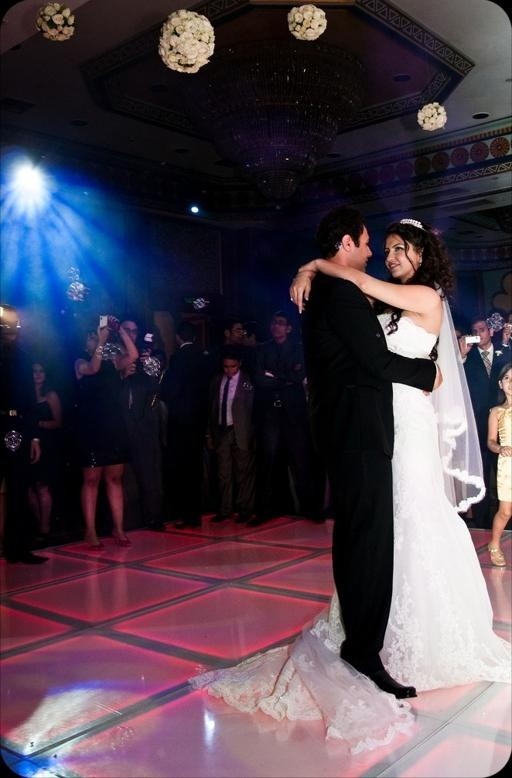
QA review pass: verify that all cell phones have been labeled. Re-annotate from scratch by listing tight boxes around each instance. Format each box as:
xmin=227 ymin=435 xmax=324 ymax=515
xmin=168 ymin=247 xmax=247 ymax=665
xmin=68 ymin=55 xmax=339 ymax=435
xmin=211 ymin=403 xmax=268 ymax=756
xmin=465 ymin=336 xmax=481 ymax=344
xmin=99 ymin=316 xmax=107 ymax=328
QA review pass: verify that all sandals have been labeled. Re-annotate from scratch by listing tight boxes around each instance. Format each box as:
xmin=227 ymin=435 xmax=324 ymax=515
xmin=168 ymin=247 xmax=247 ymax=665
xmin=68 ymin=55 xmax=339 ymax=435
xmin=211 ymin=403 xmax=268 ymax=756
xmin=486 ymin=541 xmax=507 ymax=569
xmin=85 ymin=530 xmax=132 ymax=548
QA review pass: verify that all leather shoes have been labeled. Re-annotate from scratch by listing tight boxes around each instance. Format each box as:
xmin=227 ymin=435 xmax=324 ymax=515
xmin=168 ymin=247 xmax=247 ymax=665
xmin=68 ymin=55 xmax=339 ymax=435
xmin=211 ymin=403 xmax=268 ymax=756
xmin=339 ymin=664 xmax=419 ymax=698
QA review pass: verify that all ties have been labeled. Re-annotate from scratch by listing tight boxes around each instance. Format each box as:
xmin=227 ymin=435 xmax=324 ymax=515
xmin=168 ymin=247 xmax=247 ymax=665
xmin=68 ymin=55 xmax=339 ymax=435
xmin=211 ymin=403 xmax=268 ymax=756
xmin=220 ymin=376 xmax=233 ymax=427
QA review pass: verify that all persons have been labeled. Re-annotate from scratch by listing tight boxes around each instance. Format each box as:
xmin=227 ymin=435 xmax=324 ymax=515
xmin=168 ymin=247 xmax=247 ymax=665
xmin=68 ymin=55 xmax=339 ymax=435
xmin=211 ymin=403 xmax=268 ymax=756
xmin=0 ymin=304 xmax=511 ymax=568
xmin=299 ymin=206 xmax=445 ymax=701
xmin=183 ymin=216 xmax=512 ymax=754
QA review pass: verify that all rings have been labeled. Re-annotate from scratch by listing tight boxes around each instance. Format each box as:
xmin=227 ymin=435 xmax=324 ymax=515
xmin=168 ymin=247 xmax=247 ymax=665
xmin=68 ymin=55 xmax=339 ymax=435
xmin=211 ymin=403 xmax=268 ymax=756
xmin=288 ymin=296 xmax=294 ymax=301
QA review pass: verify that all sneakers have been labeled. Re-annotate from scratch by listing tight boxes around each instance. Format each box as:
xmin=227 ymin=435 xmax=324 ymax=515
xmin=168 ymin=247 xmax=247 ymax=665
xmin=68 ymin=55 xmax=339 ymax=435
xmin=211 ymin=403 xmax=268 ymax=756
xmin=174 ymin=509 xmax=271 ymax=531
xmin=6 ymin=551 xmax=52 ymax=566
